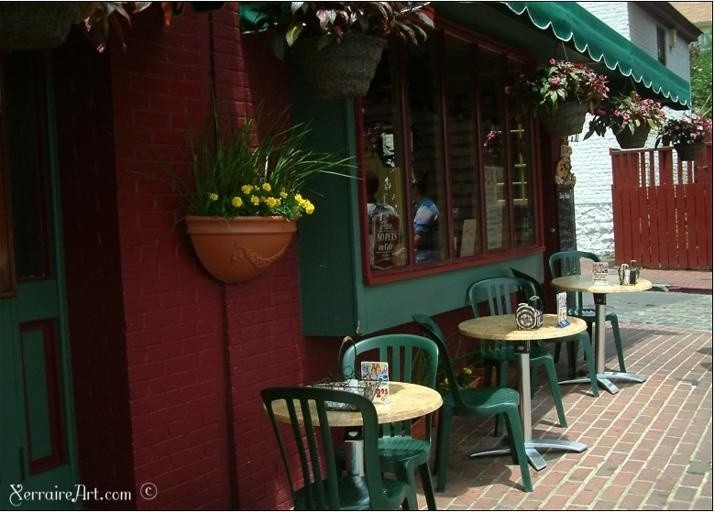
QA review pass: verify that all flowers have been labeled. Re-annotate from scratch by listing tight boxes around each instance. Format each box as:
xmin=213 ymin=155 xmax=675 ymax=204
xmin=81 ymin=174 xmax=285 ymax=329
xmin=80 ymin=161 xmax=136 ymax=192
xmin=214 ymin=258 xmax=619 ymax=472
xmin=581 ymin=85 xmax=668 ymax=141
xmin=121 ymin=66 xmax=368 ymax=221
xmin=498 ymin=55 xmax=610 ymax=120
xmin=653 ymin=111 xmax=711 ymax=151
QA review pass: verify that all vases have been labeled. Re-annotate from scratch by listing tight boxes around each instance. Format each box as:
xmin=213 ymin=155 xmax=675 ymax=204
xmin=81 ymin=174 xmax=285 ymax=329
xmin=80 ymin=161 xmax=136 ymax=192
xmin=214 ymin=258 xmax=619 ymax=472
xmin=673 ymin=139 xmax=704 ymax=161
xmin=607 ymin=117 xmax=653 ymax=149
xmin=538 ymin=98 xmax=589 ymax=134
xmin=182 ymin=212 xmax=300 ymax=285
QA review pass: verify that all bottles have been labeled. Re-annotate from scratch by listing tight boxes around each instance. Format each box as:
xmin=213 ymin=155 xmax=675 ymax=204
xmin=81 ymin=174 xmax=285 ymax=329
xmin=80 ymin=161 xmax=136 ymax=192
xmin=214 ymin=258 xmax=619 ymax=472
xmin=617 ymin=259 xmax=639 ymax=284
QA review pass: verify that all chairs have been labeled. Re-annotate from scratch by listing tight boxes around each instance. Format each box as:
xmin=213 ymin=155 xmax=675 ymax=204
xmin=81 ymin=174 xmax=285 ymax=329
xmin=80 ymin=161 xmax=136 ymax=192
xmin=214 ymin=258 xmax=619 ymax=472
xmin=255 ymin=246 xmax=626 ymax=510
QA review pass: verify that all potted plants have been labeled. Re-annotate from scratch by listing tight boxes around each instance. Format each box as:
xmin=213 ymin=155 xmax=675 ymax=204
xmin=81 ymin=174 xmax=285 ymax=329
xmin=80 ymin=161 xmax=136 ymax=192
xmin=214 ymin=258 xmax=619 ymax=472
xmin=239 ymin=1 xmax=439 ymax=101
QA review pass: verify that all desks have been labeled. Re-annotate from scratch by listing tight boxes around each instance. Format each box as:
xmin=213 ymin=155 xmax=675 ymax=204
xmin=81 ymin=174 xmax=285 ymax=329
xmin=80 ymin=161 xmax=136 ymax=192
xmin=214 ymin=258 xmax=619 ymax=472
xmin=551 ymin=273 xmax=653 ymax=394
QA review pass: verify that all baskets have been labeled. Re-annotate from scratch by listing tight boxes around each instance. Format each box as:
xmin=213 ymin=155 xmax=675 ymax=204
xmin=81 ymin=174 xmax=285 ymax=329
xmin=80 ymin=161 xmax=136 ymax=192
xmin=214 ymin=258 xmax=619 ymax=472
xmin=312 ymin=337 xmax=381 ymax=411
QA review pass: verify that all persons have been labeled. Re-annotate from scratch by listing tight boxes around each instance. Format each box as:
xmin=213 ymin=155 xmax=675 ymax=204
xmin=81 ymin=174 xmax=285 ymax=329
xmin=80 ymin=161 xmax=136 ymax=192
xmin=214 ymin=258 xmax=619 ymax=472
xmin=366 ymin=171 xmax=403 ymax=271
xmin=370 ymin=364 xmax=381 ymax=372
xmin=412 ymin=168 xmax=440 ymax=263
